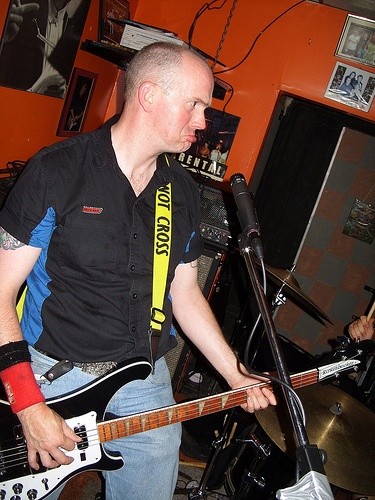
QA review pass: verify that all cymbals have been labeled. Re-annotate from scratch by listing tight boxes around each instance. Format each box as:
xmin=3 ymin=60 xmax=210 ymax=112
xmin=238 ymin=244 xmax=336 ymax=329
xmin=255 ymin=369 xmax=375 ymax=497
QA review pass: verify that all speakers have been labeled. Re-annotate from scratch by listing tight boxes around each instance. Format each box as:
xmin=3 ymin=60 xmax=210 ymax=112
xmin=163 ymin=248 xmax=225 ymax=398
xmin=193 ymin=182 xmax=238 ymax=248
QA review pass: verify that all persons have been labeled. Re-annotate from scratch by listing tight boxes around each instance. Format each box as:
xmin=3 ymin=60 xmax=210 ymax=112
xmin=0 ymin=42 xmax=278 ymax=500
xmin=348 ymin=314 xmax=375 ymax=343
xmin=194 ymin=141 xmax=228 ymax=164
xmin=0 ymin=0 xmax=128 ymax=131
xmin=330 ymin=30 xmax=375 ymax=104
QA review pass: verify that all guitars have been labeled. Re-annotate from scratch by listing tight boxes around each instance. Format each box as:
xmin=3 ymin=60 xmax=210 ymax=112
xmin=0 ymin=356 xmax=361 ymax=500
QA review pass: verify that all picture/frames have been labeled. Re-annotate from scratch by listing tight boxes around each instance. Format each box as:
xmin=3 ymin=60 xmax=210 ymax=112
xmin=324 ymin=61 xmax=375 ymax=113
xmin=57 ymin=67 xmax=98 ymax=136
xmin=335 ymin=13 xmax=375 ymax=68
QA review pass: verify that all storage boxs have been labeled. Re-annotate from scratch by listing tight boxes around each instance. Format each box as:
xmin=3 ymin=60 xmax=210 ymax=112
xmin=163 ymin=248 xmax=222 ymax=393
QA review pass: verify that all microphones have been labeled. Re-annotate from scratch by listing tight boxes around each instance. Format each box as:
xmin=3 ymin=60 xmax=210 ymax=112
xmin=229 ymin=172 xmax=263 ymax=259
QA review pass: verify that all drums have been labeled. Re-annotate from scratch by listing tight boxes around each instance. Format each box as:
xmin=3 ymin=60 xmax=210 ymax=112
xmin=223 ymin=423 xmax=338 ymax=500
